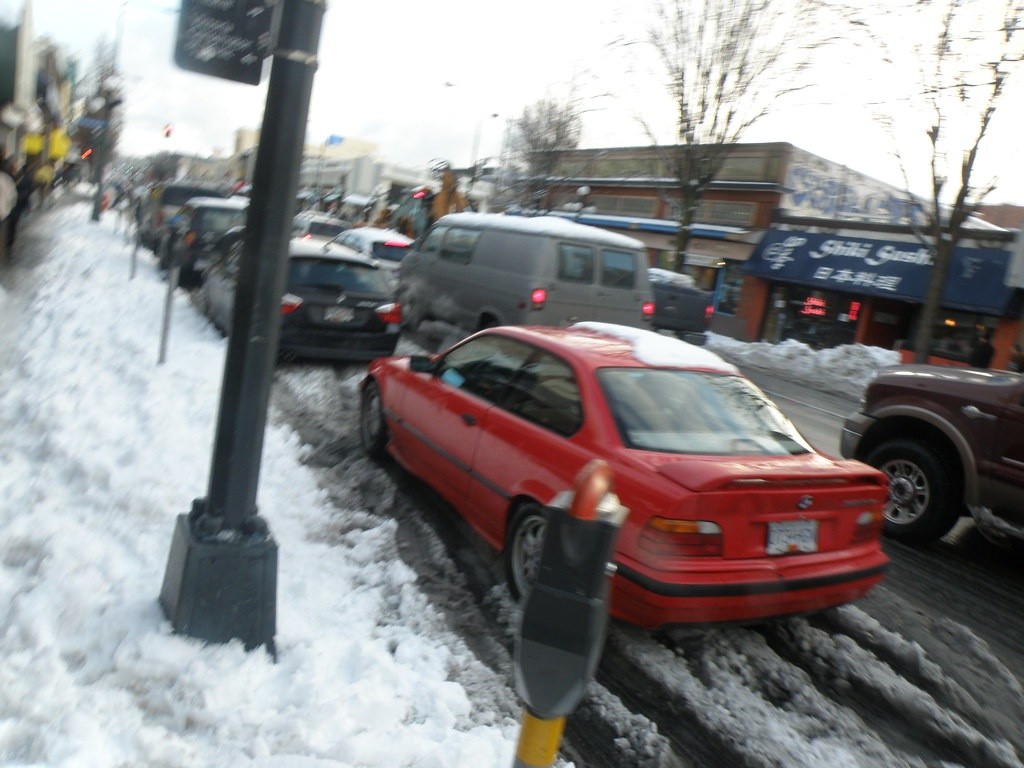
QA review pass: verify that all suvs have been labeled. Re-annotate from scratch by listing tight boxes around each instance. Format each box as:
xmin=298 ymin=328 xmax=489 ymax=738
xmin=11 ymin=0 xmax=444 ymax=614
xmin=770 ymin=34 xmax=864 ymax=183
xmin=838 ymin=363 xmax=1023 ymax=557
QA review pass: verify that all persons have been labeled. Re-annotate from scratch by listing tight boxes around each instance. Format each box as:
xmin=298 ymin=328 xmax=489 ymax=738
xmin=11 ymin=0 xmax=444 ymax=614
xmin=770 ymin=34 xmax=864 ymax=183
xmin=969 ymin=334 xmax=994 ymax=368
xmin=1008 ymin=342 xmax=1024 ymax=373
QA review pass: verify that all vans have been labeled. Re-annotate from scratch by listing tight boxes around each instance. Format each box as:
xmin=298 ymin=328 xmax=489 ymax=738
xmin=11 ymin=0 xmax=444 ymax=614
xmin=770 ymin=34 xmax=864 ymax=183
xmin=397 ymin=214 xmax=656 ymax=346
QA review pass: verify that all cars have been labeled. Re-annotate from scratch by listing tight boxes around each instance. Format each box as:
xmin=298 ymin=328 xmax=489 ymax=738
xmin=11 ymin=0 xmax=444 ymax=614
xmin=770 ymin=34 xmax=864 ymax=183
xmin=644 ymin=269 xmax=715 ymax=337
xmin=108 ymin=168 xmax=414 ymax=363
xmin=359 ymin=324 xmax=890 ymax=633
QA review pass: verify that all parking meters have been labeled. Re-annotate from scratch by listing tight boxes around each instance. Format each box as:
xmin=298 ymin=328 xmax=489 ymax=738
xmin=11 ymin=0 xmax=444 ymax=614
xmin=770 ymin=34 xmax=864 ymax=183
xmin=508 ymin=456 xmax=630 ymax=768
